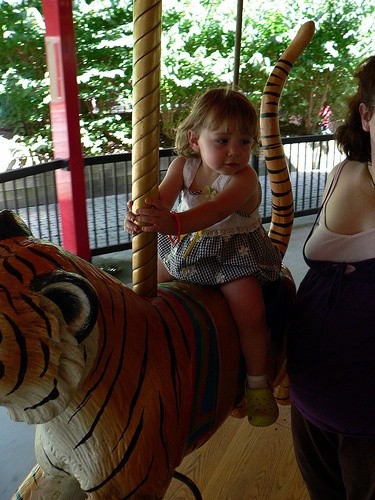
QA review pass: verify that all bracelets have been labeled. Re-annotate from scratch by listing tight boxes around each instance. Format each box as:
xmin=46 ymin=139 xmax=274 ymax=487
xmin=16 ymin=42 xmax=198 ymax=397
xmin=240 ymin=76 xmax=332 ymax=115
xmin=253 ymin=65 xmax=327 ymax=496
xmin=167 ymin=211 xmax=182 ymax=246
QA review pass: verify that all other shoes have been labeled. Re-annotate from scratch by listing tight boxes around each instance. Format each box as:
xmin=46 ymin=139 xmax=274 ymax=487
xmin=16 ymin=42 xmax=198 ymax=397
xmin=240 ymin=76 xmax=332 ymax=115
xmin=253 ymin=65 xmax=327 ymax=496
xmin=245 ymin=379 xmax=279 ymax=427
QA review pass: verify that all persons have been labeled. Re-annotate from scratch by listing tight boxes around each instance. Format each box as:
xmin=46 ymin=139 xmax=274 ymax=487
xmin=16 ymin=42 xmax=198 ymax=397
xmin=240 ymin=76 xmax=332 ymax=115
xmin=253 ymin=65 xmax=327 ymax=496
xmin=282 ymin=54 xmax=375 ymax=500
xmin=317 ymin=102 xmax=332 ymax=132
xmin=122 ymin=89 xmax=281 ymax=427
